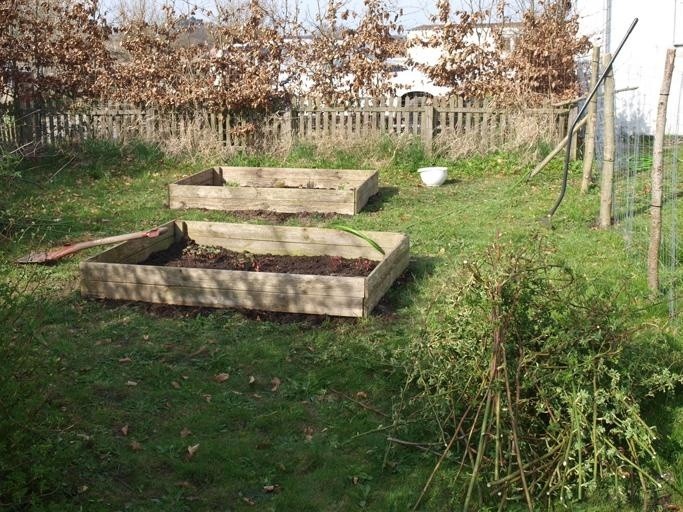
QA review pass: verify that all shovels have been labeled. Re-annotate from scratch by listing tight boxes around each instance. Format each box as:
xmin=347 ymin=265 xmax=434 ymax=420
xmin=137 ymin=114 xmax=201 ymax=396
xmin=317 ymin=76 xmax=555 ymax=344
xmin=15 ymin=226 xmax=160 ymax=263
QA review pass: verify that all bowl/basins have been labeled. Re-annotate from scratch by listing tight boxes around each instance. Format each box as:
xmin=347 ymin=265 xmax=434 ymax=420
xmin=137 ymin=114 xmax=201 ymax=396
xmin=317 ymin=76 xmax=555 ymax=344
xmin=416 ymin=165 xmax=448 ymax=187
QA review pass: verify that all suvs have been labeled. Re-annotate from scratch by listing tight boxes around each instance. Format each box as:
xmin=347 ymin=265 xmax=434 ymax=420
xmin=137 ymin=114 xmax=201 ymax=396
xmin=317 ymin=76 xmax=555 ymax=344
xmin=213 ymin=36 xmax=455 ymax=104
